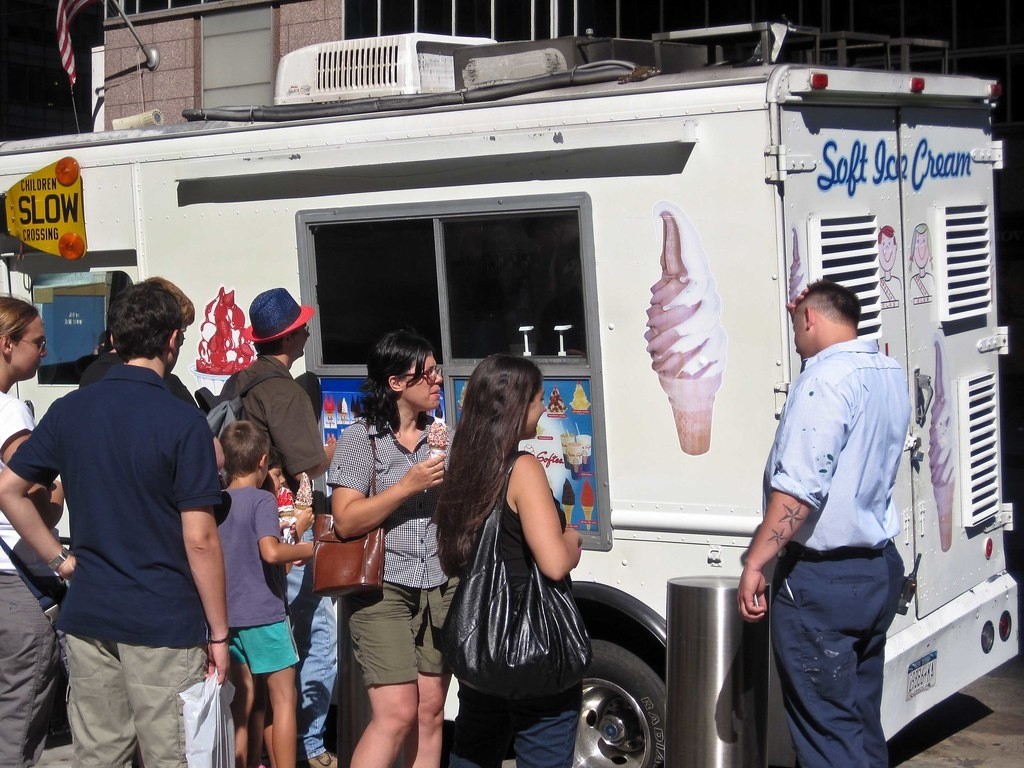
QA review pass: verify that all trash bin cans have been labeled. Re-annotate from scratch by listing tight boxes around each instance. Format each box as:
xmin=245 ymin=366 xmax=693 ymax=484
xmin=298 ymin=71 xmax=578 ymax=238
xmin=664 ymin=575 xmax=770 ymax=768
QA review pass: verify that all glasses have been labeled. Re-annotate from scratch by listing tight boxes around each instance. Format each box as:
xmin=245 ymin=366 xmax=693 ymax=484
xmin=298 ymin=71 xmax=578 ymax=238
xmin=398 ymin=368 xmax=442 ymax=381
xmin=9 ymin=334 xmax=46 ymax=353
xmin=289 ymin=326 xmax=309 ymax=335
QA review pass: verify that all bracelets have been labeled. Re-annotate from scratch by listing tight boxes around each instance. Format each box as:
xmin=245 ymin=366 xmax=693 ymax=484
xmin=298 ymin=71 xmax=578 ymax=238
xmin=48 ymin=546 xmax=70 ymax=571
xmin=208 ymin=634 xmax=230 ymax=644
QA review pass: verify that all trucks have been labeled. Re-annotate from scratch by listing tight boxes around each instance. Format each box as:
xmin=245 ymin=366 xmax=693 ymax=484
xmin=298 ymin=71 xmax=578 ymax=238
xmin=0 ymin=30 xmax=1021 ymax=768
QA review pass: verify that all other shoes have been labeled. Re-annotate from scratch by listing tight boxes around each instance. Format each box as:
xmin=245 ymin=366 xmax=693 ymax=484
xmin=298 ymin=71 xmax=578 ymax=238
xmin=295 ymin=746 xmax=337 ymax=768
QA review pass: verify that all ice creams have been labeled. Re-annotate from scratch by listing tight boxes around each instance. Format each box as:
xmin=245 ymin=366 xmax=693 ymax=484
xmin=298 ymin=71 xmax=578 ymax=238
xmin=644 ymin=200 xmax=727 ymax=454
xmin=561 ymin=478 xmax=594 ymax=531
xmin=276 ymin=487 xmax=294 ymax=518
xmin=324 ymin=395 xmax=362 ymax=429
xmin=548 ymin=381 xmax=591 ymax=414
xmin=427 ymin=422 xmax=448 ymax=451
xmin=295 ymin=472 xmax=313 ymax=511
xmin=928 ymin=332 xmax=957 ymax=552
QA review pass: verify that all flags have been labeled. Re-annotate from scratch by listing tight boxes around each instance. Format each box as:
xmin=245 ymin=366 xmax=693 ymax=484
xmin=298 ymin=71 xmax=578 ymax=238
xmin=56 ymin=0 xmax=93 ymax=87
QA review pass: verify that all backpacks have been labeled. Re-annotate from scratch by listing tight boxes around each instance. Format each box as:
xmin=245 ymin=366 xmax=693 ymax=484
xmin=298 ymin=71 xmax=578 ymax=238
xmin=195 ymin=372 xmax=291 ymax=485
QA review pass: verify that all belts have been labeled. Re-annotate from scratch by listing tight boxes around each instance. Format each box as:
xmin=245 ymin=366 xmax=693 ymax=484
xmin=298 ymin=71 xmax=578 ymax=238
xmin=776 ymin=542 xmax=825 ymax=563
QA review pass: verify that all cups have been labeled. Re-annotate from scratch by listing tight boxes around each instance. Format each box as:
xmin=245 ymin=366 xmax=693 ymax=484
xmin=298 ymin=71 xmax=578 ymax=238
xmin=560 ymin=435 xmax=592 ymax=481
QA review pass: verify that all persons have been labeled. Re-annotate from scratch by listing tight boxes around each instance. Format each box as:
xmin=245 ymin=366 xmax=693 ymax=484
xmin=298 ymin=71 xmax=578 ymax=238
xmin=736 ymin=281 xmax=910 ymax=768
xmin=0 ymin=276 xmax=317 ymax=768
xmin=326 ymin=330 xmax=456 ymax=768
xmin=435 ymin=351 xmax=584 ymax=768
xmin=207 ymin=288 xmax=339 ymax=768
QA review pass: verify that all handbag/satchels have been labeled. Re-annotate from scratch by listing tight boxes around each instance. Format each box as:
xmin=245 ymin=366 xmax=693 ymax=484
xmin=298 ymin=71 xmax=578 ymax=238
xmin=442 ymin=452 xmax=593 ymax=695
xmin=178 ymin=666 xmax=236 ymax=768
xmin=313 ymin=421 xmax=385 ymax=595
xmin=48 ymin=604 xmax=68 ymax=678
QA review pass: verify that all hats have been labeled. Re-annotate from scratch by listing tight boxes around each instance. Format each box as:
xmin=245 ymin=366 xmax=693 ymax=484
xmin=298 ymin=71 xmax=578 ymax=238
xmin=244 ymin=287 xmax=315 ymax=342
